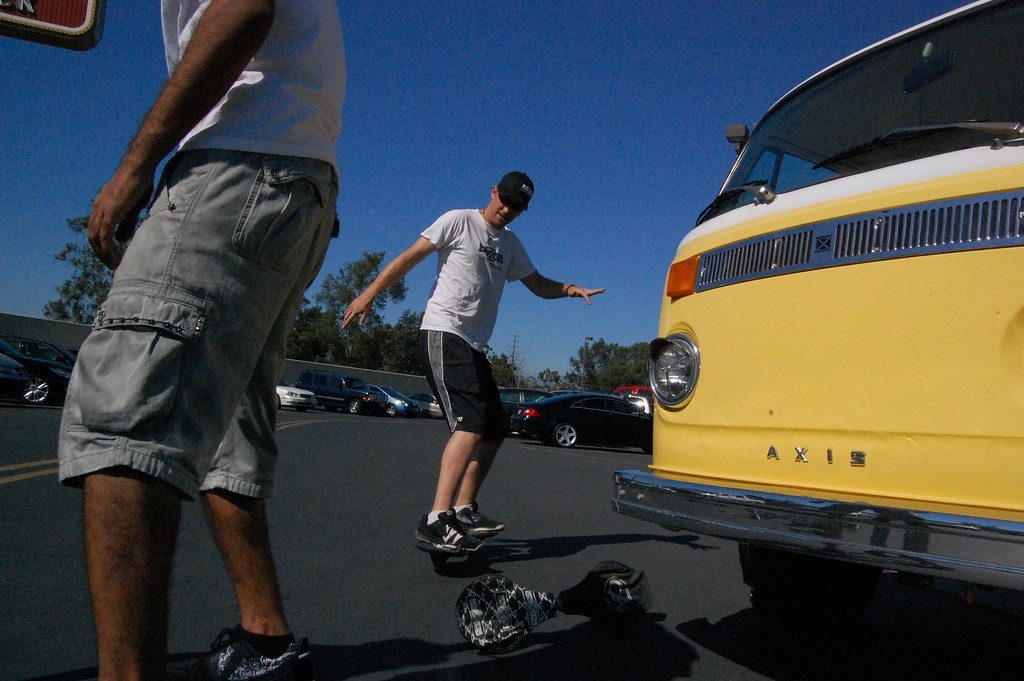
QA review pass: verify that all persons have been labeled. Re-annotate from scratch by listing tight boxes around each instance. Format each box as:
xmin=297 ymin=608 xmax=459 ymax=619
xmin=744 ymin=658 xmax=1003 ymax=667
xmin=60 ymin=0 xmax=345 ymax=681
xmin=342 ymin=170 xmax=606 ymax=551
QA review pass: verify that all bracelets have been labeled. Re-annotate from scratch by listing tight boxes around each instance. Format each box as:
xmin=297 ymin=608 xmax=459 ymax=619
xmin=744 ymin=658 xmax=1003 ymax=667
xmin=566 ymin=285 xmax=577 ymax=296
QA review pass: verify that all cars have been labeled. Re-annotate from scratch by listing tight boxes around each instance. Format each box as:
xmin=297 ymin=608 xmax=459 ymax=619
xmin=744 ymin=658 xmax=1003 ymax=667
xmin=353 ymin=384 xmax=424 ymax=419
xmin=510 ymin=391 xmax=652 ymax=452
xmin=404 ymin=390 xmax=447 ymax=421
xmin=499 ymin=388 xmax=562 ymax=426
xmin=276 ymin=380 xmax=319 ymax=412
xmin=0 ymin=336 xmax=80 ymax=405
xmin=612 ymin=386 xmax=654 ymax=413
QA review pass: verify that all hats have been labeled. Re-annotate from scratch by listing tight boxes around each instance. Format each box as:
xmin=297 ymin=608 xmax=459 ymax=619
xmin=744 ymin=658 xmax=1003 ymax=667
xmin=497 ymin=171 xmax=535 ymax=206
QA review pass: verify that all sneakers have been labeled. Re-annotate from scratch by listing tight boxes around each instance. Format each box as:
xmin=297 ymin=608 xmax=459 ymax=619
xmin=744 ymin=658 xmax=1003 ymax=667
xmin=165 ymin=624 xmax=313 ymax=681
xmin=415 ymin=508 xmax=485 ymax=552
xmin=456 ymin=501 xmax=506 ymax=532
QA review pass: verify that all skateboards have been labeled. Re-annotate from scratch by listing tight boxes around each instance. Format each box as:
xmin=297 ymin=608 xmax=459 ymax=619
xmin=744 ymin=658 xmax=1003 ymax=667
xmin=418 ymin=533 xmax=492 ymax=575
xmin=453 ymin=559 xmax=654 ymax=654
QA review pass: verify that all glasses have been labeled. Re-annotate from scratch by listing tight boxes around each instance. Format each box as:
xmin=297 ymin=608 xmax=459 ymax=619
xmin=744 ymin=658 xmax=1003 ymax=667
xmin=500 ymin=194 xmax=523 ymax=214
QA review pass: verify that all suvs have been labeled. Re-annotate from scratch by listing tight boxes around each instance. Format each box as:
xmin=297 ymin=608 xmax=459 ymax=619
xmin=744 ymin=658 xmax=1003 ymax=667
xmin=296 ymin=368 xmax=389 ymax=416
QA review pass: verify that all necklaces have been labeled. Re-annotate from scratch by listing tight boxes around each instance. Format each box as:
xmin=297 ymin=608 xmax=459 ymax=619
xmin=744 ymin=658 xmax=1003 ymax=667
xmin=483 ymin=208 xmax=500 ymax=245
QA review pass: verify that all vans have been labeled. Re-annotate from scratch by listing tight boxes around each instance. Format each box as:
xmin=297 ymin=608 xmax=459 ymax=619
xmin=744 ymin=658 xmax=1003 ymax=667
xmin=613 ymin=0 xmax=1024 ymax=627
